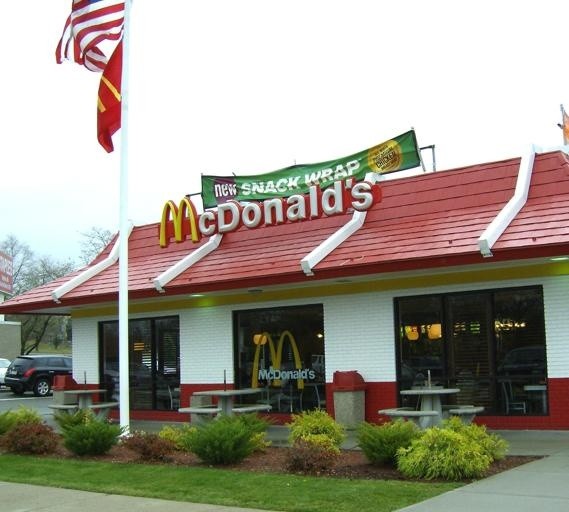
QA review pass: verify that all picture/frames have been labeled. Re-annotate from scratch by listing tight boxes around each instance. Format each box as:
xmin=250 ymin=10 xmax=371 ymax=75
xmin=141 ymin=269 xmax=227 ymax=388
xmin=523 ymin=385 xmax=547 ymax=415
xmin=64 ymin=389 xmax=106 ymax=413
xmin=193 ymin=387 xmax=264 ymax=418
xmin=400 ymin=388 xmax=461 ymax=428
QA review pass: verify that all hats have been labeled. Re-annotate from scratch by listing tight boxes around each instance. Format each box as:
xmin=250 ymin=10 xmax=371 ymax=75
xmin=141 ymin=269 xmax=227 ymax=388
xmin=407 ymin=355 xmax=474 ymax=406
xmin=0 ymin=357 xmax=12 ymax=387
xmin=125 ymin=361 xmax=151 ymax=385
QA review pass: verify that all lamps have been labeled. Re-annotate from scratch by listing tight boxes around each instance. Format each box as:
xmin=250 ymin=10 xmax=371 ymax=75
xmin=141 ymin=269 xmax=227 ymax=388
xmin=312 ymin=383 xmax=326 ymax=410
xmin=168 ymin=385 xmax=179 ymax=410
xmin=509 ymin=380 xmax=519 ymax=400
xmin=502 ymin=382 xmax=527 ymax=414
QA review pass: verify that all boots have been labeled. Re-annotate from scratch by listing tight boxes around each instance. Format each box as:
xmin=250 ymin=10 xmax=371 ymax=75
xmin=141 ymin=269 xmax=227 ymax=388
xmin=496 ymin=342 xmax=545 ymax=403
xmin=3 ymin=353 xmax=72 ymax=398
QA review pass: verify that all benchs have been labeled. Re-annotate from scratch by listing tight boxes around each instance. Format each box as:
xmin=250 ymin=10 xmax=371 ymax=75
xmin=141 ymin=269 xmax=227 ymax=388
xmin=54 ymin=0 xmax=125 ymax=153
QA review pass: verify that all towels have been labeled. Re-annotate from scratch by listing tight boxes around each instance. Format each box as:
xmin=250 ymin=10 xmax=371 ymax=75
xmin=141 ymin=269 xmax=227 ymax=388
xmin=51 ymin=375 xmax=77 ymax=413
xmin=331 ymin=370 xmax=367 ymax=431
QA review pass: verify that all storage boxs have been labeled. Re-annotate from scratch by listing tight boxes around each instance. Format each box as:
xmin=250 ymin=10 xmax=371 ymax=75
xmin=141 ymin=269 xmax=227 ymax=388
xmin=47 ymin=402 xmax=119 ymax=422
xmin=378 ymin=405 xmax=485 ymax=431
xmin=178 ymin=403 xmax=272 ymax=425
